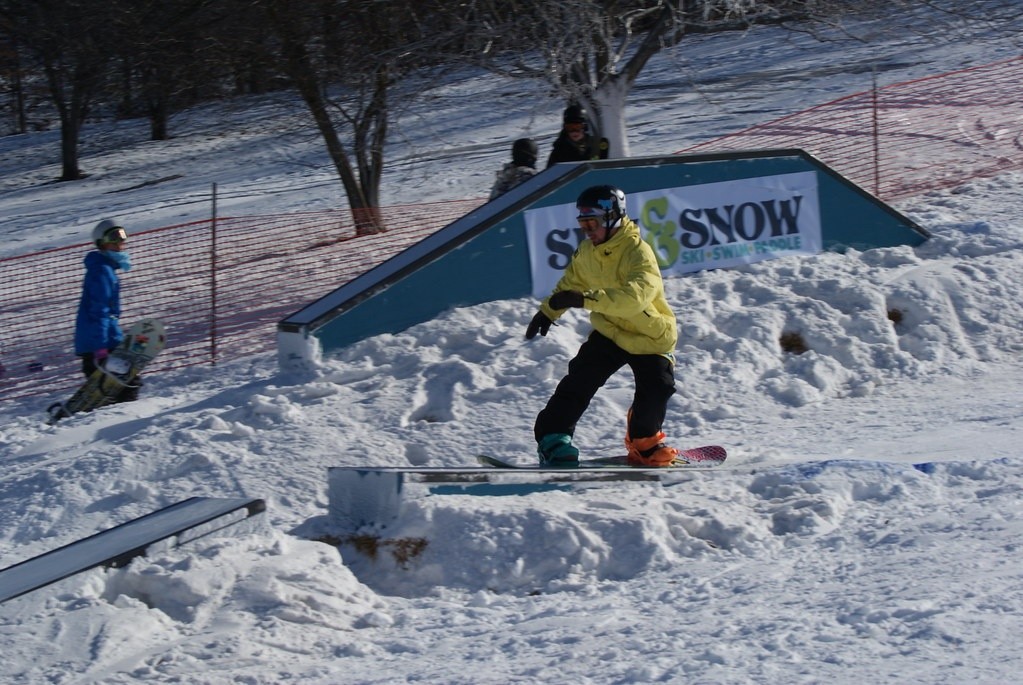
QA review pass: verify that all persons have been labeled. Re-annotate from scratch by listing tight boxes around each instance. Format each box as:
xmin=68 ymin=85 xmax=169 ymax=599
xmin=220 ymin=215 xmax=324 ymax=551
xmin=526 ymin=185 xmax=678 ymax=470
xmin=489 ymin=138 xmax=539 ymax=201
xmin=75 ymin=220 xmax=132 ymax=380
xmin=546 ymin=105 xmax=609 ymax=168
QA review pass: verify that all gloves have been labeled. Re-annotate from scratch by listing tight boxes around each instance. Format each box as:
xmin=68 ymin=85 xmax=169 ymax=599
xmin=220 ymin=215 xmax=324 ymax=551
xmin=526 ymin=312 xmax=552 ymax=339
xmin=94 ymin=348 xmax=108 ymax=359
xmin=548 ymin=289 xmax=584 ymax=310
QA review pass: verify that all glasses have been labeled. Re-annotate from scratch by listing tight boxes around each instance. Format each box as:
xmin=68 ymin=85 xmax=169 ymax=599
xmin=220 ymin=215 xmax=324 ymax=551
xmin=577 ymin=214 xmax=607 ymax=232
xmin=103 ymin=228 xmax=127 ymax=243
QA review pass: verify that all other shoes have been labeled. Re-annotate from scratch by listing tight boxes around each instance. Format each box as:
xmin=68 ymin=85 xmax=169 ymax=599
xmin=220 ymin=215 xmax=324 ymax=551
xmin=623 ymin=405 xmax=678 ymax=466
xmin=538 ymin=435 xmax=579 ymax=468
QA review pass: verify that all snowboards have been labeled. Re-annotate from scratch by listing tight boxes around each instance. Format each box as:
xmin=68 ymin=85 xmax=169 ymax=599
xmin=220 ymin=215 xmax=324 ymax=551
xmin=477 ymin=443 xmax=730 ymax=473
xmin=44 ymin=316 xmax=170 ymax=427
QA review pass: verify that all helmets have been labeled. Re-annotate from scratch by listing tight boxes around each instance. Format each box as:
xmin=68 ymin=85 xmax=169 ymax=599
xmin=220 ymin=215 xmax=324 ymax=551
xmin=576 ymin=185 xmax=625 ymax=229
xmin=563 ymin=105 xmax=589 ymax=131
xmin=512 ymin=138 xmax=537 ymax=164
xmin=564 ymin=122 xmax=585 ymax=132
xmin=92 ymin=219 xmax=124 ymax=242
